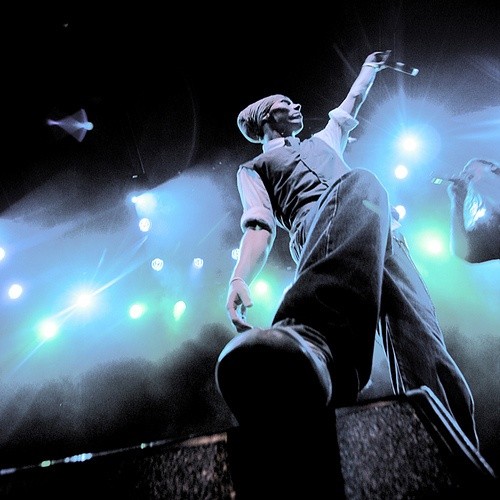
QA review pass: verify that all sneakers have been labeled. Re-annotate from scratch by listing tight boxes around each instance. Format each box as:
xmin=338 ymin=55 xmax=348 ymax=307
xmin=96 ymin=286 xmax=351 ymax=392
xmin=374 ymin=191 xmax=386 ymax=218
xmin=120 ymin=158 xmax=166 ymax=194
xmin=216 ymin=318 xmax=334 ymax=423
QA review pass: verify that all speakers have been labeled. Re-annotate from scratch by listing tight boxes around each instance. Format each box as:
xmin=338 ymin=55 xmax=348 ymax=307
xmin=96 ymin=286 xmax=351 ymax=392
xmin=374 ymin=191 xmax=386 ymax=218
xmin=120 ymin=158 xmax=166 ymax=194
xmin=0 ymin=387 xmax=500 ymax=500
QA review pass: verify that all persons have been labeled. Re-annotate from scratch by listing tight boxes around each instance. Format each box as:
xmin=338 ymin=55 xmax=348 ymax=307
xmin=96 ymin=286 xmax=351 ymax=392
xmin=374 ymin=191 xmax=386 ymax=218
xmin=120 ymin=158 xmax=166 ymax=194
xmin=215 ymin=49 xmax=480 ymax=452
xmin=448 ymin=159 xmax=500 ymax=264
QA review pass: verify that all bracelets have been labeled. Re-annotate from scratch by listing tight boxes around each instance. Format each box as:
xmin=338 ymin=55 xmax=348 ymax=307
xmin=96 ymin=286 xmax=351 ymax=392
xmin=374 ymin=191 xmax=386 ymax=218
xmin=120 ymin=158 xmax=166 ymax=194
xmin=362 ymin=63 xmax=379 ymax=72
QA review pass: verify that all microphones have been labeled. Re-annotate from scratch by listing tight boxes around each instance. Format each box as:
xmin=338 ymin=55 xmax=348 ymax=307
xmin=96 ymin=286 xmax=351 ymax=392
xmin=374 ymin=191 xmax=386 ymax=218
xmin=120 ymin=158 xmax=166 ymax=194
xmin=376 ymin=53 xmax=419 ymax=76
xmin=432 ymin=174 xmax=460 ymax=184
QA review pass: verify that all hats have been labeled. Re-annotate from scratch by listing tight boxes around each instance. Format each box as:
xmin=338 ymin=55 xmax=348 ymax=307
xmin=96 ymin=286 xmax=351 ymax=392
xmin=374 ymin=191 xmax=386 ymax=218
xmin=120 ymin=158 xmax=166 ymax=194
xmin=237 ymin=95 xmax=285 ymax=143
